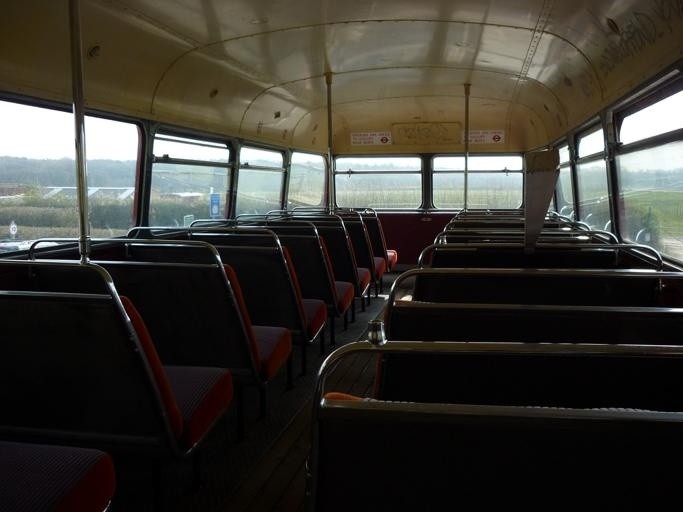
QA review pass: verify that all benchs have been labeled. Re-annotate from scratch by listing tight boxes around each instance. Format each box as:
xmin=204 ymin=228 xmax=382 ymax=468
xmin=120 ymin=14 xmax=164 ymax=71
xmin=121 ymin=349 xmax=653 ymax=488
xmin=0 ymin=204 xmax=683 ymax=512
